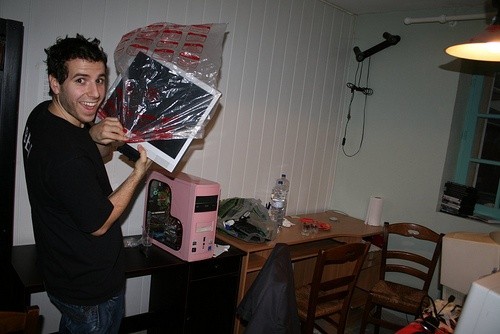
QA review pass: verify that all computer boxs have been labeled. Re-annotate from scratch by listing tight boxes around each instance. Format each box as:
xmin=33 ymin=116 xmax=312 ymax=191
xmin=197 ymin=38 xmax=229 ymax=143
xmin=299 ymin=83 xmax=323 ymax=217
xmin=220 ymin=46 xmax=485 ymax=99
xmin=143 ymin=164 xmax=222 ymax=262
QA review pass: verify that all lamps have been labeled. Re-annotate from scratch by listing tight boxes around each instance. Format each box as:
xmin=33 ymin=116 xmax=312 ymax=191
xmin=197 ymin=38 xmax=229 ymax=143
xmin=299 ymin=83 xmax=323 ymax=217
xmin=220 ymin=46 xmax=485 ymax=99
xmin=443 ymin=14 xmax=500 ymax=63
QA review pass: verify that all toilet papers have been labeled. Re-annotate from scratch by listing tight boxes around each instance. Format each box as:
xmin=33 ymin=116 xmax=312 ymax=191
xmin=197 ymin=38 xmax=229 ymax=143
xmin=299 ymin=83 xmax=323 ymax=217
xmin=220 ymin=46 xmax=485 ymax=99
xmin=364 ymin=196 xmax=384 ymax=226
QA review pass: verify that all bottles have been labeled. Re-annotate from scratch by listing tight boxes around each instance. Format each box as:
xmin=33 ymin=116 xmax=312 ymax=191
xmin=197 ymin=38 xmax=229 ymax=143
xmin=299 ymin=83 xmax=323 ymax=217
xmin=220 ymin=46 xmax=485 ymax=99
xmin=271 ymin=174 xmax=290 ymax=233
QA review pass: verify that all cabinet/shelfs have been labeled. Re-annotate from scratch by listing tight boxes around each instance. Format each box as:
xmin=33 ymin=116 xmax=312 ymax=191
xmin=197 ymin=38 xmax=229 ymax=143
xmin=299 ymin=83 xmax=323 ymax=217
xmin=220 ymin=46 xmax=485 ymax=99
xmin=11 ymin=236 xmax=247 ymax=334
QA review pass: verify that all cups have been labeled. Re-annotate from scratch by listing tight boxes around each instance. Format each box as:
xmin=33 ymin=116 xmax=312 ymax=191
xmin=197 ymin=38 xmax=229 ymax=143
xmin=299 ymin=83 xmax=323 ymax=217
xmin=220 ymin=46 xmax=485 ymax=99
xmin=302 ymin=221 xmax=311 ymax=237
xmin=142 ymin=223 xmax=152 ymax=247
xmin=311 ymin=219 xmax=319 ymax=234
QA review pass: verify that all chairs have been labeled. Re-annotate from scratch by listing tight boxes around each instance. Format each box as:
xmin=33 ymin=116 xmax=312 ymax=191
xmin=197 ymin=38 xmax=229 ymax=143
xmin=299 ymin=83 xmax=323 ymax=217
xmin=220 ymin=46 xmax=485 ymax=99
xmin=295 ymin=241 xmax=371 ymax=334
xmin=360 ymin=221 xmax=445 ymax=334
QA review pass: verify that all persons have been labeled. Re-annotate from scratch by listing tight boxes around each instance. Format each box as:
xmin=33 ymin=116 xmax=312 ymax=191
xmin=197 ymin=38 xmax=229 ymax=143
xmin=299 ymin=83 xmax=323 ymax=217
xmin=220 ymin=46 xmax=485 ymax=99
xmin=22 ymin=32 xmax=153 ymax=334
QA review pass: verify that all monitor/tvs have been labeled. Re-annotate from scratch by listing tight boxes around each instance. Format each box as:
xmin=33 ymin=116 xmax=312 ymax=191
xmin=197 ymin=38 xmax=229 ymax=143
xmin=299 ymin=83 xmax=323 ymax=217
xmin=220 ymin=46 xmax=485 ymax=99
xmin=93 ymin=48 xmax=222 ymax=172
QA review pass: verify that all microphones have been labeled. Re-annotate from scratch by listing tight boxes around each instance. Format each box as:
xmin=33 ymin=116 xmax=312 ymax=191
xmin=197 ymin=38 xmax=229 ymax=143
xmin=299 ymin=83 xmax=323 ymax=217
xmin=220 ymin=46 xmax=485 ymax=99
xmin=438 ymin=295 xmax=455 ymax=315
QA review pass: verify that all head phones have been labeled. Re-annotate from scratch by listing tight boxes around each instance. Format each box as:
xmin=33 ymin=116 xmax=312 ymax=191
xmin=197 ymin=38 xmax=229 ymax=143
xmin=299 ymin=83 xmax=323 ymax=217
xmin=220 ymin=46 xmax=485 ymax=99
xmin=416 ymin=295 xmax=440 ymax=333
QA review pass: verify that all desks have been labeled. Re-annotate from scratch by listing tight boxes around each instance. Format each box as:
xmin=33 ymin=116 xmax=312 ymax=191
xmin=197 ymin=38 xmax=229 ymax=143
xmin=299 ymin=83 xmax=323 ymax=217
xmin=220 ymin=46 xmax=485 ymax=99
xmin=216 ymin=211 xmax=384 ymax=334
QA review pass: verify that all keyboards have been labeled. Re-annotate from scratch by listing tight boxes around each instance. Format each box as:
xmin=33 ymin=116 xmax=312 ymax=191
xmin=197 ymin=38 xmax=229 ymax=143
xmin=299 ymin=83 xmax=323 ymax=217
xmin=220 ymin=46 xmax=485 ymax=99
xmin=230 ymin=220 xmax=266 ymax=243
